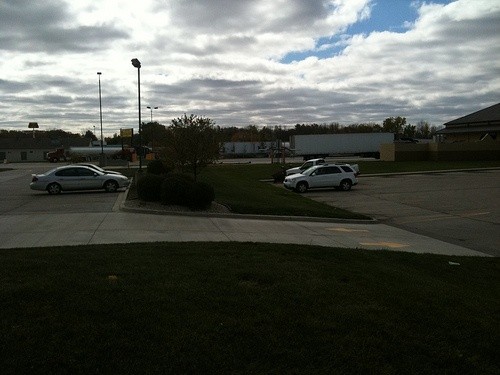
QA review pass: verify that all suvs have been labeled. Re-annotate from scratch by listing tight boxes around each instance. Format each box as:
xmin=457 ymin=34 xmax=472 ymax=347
xmin=283 ymin=162 xmax=359 ymax=192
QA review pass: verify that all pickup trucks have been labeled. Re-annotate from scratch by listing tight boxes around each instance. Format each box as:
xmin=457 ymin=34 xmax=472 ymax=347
xmin=286 ymin=158 xmax=360 ymax=178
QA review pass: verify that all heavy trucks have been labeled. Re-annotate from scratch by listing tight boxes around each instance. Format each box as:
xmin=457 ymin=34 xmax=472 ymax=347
xmin=46 ymin=146 xmax=125 ymax=162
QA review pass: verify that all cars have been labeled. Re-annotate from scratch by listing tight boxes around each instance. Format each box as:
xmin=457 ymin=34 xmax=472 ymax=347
xmin=71 ymin=162 xmax=122 ymax=176
xmin=29 ymin=165 xmax=130 ymax=194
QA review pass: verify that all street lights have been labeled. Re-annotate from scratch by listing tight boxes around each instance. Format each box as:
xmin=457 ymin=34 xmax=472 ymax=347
xmin=148 ymin=104 xmax=159 ymax=158
xmin=96 ymin=71 xmax=107 ymax=164
xmin=131 ymin=58 xmax=142 ymax=173
